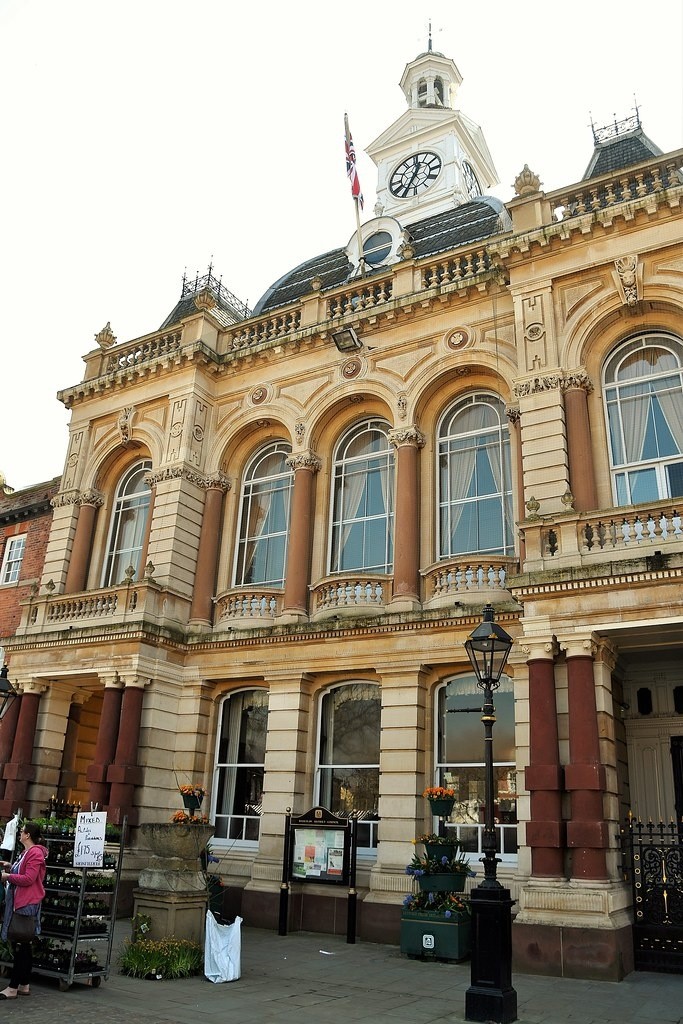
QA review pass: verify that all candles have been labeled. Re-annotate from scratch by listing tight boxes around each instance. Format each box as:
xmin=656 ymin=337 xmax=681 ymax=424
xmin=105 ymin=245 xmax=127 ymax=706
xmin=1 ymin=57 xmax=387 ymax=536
xmin=78 ymin=801 xmax=81 ymax=808
xmin=55 ymin=799 xmax=58 ymax=804
xmin=638 ymin=816 xmax=641 ymax=822
xmin=659 ymin=817 xmax=663 ymax=823
xmin=628 ymin=812 xmax=632 ymax=827
xmin=648 ymin=816 xmax=652 ymax=823
xmin=66 ymin=800 xmax=69 ymax=806
xmin=670 ymin=816 xmax=674 ymax=823
xmin=61 ymin=800 xmax=64 ymax=806
xmin=73 ymin=800 xmax=75 ymax=807
xmin=51 ymin=794 xmax=54 ymax=806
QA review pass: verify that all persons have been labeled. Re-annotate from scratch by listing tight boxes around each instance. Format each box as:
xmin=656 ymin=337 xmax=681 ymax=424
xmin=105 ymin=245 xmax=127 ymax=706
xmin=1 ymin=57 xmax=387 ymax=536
xmin=0 ymin=822 xmax=48 ymax=1001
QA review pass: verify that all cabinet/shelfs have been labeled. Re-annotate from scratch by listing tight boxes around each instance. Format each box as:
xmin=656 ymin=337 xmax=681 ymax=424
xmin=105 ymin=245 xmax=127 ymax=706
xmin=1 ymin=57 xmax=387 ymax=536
xmin=0 ymin=809 xmax=128 ymax=990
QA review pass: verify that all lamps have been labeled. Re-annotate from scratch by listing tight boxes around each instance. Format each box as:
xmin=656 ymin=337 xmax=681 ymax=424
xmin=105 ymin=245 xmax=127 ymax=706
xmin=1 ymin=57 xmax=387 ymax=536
xmin=331 ymin=327 xmax=363 ymax=354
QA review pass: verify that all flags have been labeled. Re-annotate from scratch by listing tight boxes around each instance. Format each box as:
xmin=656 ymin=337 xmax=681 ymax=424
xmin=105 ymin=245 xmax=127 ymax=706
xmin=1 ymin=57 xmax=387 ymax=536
xmin=344 ymin=113 xmax=364 ymax=211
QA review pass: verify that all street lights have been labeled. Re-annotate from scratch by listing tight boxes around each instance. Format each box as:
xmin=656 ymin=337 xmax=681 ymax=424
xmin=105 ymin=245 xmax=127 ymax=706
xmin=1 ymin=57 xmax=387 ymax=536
xmin=462 ymin=601 xmax=522 ymax=1023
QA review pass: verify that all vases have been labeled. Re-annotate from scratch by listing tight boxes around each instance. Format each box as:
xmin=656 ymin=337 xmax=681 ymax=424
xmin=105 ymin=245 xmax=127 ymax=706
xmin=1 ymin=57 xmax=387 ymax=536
xmin=422 ymin=842 xmax=462 ymax=861
xmin=183 ymin=794 xmax=204 ymax=808
xmin=427 ymin=798 xmax=456 ymax=816
xmin=402 ymin=908 xmax=471 ymax=923
xmin=413 ymin=873 xmax=467 ymax=892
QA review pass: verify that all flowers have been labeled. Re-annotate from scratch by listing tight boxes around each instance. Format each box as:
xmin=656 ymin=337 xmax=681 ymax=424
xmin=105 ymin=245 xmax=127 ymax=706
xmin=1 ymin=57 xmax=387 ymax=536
xmin=404 ymin=853 xmax=477 ymax=879
xmin=168 ymin=810 xmax=211 ymax=824
xmin=181 ymin=785 xmax=208 ymax=796
xmin=422 ymin=786 xmax=456 ymax=800
xmin=411 ymin=832 xmax=462 ymax=846
xmin=402 ymin=892 xmax=470 ymax=912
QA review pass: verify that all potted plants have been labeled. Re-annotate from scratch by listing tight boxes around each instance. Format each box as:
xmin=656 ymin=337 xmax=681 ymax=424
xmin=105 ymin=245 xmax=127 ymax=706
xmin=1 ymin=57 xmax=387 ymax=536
xmin=0 ymin=818 xmax=122 ymax=973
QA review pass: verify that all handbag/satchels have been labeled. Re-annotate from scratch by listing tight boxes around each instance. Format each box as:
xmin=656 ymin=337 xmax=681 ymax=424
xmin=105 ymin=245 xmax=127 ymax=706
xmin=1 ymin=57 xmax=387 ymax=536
xmin=6 ymin=911 xmax=35 ymax=941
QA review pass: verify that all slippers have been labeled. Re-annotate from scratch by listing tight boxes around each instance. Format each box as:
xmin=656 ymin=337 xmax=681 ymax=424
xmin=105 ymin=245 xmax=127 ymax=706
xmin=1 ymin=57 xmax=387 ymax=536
xmin=0 ymin=993 xmax=18 ymax=1000
xmin=17 ymin=987 xmax=31 ymax=996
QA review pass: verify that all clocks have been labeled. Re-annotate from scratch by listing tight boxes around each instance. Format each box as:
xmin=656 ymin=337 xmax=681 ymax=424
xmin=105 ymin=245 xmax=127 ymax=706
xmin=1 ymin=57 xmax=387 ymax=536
xmin=388 ymin=149 xmax=442 ymax=200
